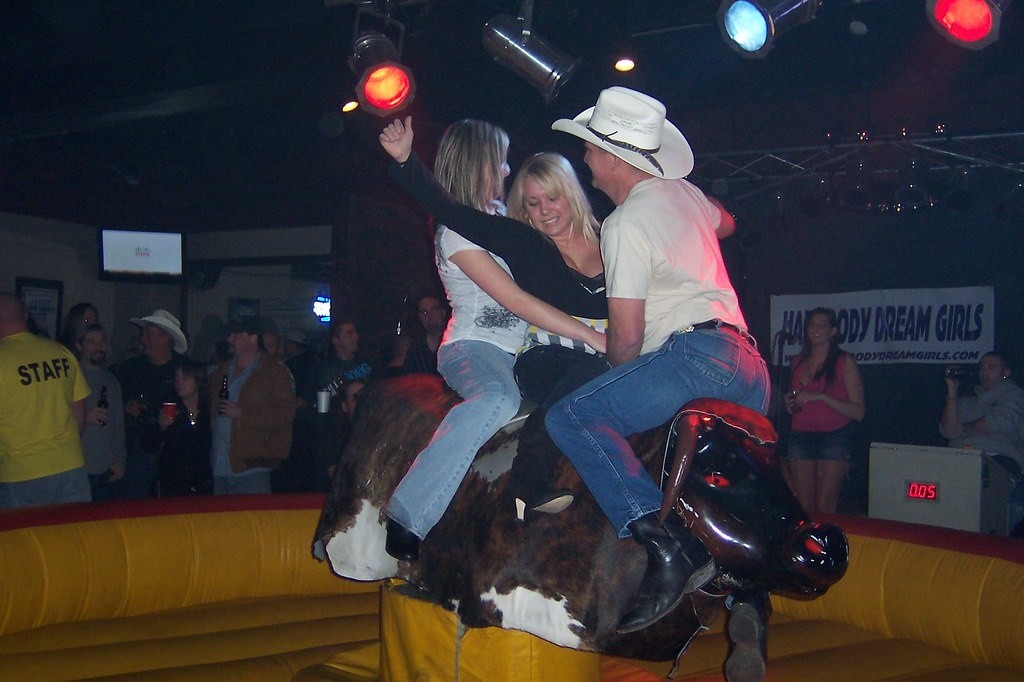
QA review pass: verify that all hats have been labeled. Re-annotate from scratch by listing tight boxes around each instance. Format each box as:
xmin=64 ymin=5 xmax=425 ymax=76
xmin=129 ymin=310 xmax=188 ymax=355
xmin=551 ymin=86 xmax=694 ymax=180
xmin=222 ymin=311 xmax=261 ymax=334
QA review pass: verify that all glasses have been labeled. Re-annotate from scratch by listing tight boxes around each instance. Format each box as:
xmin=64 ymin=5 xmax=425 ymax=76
xmin=417 ymin=306 xmax=441 ymax=317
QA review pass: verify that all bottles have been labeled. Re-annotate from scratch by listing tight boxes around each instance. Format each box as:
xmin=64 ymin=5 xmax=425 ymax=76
xmin=946 ymin=368 xmax=978 ymax=381
xmin=97 ymin=385 xmax=108 ymax=425
xmin=218 ymin=375 xmax=229 ymax=414
xmin=136 ymin=394 xmax=147 ymax=426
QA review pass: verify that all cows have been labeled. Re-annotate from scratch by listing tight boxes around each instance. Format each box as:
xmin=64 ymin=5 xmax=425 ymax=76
xmin=319 ymin=371 xmax=849 ymax=663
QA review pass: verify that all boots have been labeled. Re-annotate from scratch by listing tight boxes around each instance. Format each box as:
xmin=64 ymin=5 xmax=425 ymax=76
xmin=509 ymin=413 xmax=574 ymax=521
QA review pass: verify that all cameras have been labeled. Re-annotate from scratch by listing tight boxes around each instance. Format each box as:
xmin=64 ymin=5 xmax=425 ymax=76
xmin=943 ymin=367 xmax=960 ymax=377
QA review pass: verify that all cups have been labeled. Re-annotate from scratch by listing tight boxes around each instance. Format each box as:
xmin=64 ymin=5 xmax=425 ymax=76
xmin=317 ymin=391 xmax=330 ymax=413
xmin=789 ymin=392 xmax=802 ymax=413
xmin=163 ymin=403 xmax=176 ymax=419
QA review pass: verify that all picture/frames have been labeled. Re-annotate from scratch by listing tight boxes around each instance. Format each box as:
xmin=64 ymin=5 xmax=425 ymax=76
xmin=15 ymin=275 xmax=64 ymax=341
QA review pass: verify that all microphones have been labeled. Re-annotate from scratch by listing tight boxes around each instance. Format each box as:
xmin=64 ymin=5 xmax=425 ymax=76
xmin=780 ymin=330 xmax=798 ymax=344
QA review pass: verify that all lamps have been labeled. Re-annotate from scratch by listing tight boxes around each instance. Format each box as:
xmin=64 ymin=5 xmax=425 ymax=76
xmin=716 ymin=0 xmax=821 ymax=60
xmin=925 ymin=0 xmax=1011 ymax=51
xmin=345 ymin=0 xmax=417 ymax=117
xmin=480 ymin=0 xmax=592 ymax=107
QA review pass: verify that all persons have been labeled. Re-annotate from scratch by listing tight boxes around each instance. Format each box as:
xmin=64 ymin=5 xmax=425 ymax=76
xmin=385 ymin=334 xmax=416 ymax=378
xmin=938 ymin=351 xmax=1024 ymax=538
xmin=378 ymin=114 xmax=611 ymax=562
xmin=316 ymin=378 xmax=381 ymax=482
xmin=317 ymin=319 xmax=373 ymax=491
xmin=0 ymin=292 xmax=93 ymax=509
xmin=403 ymin=293 xmax=450 ymax=376
xmin=783 ymin=306 xmax=866 ymax=514
xmin=544 ymin=85 xmax=773 ymax=682
xmin=58 ymin=302 xmax=324 ymax=501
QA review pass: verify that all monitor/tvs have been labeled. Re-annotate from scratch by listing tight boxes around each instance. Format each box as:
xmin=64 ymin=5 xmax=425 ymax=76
xmin=98 ymin=227 xmax=187 ymax=284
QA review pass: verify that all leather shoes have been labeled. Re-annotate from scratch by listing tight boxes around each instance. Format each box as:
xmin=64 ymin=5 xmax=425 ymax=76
xmin=615 ymin=507 xmax=716 ymax=634
xmin=385 ymin=515 xmax=420 ymax=562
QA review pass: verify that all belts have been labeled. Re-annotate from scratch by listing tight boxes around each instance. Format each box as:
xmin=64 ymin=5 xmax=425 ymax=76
xmin=679 ymin=319 xmax=755 ymax=347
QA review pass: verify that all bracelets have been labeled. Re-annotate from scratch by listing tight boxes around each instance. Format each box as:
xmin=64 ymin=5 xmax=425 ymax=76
xmin=396 ymin=161 xmax=406 ymax=165
